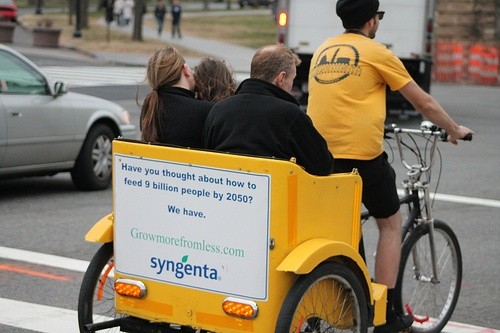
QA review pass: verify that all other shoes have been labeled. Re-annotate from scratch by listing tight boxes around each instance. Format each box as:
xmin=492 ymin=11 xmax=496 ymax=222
xmin=373 ymin=312 xmax=413 ymax=333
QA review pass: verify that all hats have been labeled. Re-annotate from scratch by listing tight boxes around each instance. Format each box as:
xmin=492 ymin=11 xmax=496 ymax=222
xmin=335 ymin=0 xmax=380 ymax=30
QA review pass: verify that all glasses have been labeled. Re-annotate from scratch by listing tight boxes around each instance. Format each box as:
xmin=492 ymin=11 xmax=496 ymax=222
xmin=375 ymin=10 xmax=386 ymax=21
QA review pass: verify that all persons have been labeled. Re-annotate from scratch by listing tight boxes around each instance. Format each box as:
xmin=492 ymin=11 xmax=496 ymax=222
xmin=154 ymin=0 xmax=166 ymax=34
xmin=171 ymin=0 xmax=181 ymax=38
xmin=101 ymin=0 xmax=135 ymax=26
xmin=139 ymin=47 xmax=213 ymax=148
xmin=194 ymin=58 xmax=235 ymax=101
xmin=203 ymin=45 xmax=335 ymax=177
xmin=306 ymin=0 xmax=474 ymax=333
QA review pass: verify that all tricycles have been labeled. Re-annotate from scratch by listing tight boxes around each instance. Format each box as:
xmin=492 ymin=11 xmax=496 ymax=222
xmin=77 ymin=120 xmax=473 ymax=333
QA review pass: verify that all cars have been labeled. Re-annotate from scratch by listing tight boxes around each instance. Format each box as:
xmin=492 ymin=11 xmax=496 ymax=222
xmin=0 ymin=0 xmax=17 ymax=22
xmin=0 ymin=45 xmax=136 ymax=192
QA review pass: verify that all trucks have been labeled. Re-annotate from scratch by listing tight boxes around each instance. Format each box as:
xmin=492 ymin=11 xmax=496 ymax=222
xmin=272 ymin=0 xmax=437 ymax=119
